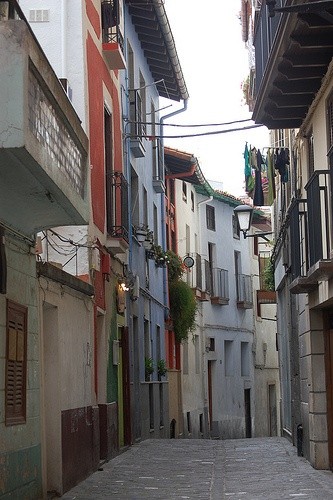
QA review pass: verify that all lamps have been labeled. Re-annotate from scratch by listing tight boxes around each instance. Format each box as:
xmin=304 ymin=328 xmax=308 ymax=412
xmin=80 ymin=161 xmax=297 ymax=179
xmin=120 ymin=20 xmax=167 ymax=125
xmin=234 ymin=204 xmax=254 ymax=236
